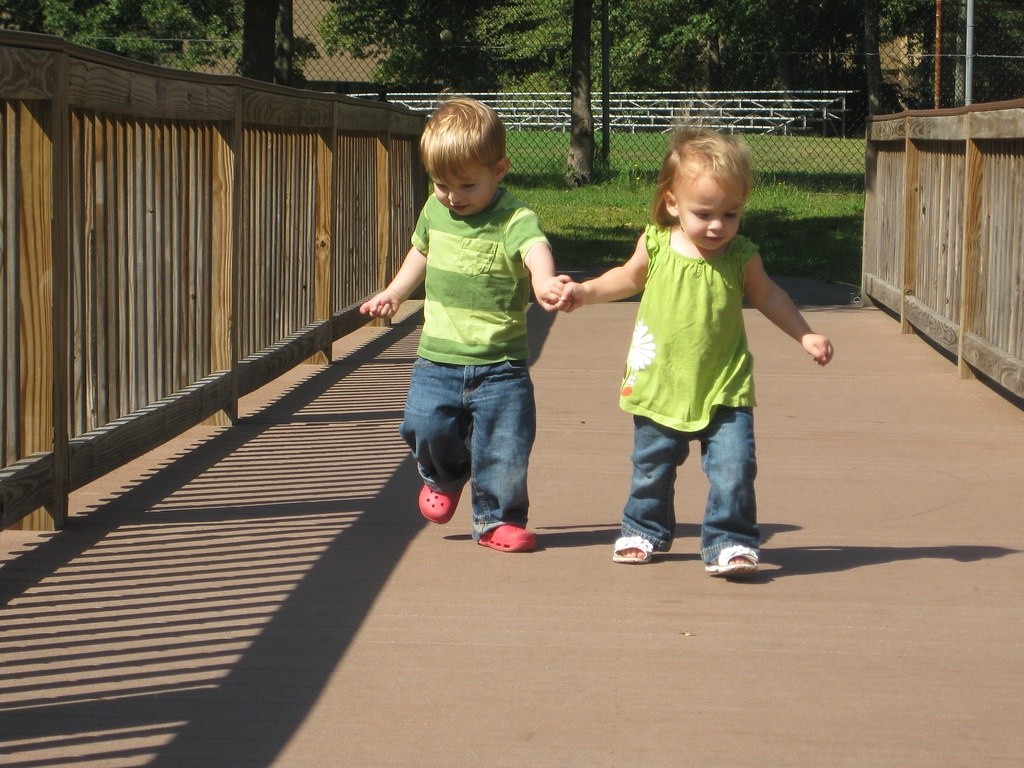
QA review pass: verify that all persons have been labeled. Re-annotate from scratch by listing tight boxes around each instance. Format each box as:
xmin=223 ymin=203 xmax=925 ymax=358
xmin=359 ymin=96 xmax=573 ymax=552
xmin=544 ymin=128 xmax=834 ymax=573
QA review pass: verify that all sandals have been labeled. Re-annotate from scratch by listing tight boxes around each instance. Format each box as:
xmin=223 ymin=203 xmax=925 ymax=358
xmin=418 ymin=481 xmax=464 ymax=525
xmin=477 ymin=525 xmax=536 ymax=552
xmin=613 ymin=532 xmax=653 ymax=565
xmin=704 ymin=545 xmax=758 ymax=575
